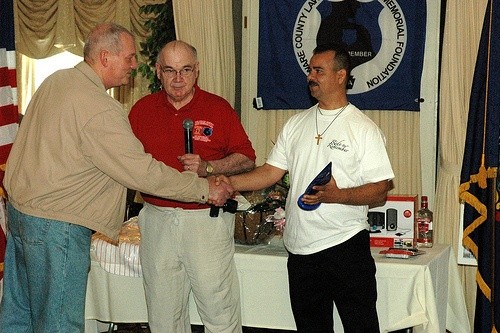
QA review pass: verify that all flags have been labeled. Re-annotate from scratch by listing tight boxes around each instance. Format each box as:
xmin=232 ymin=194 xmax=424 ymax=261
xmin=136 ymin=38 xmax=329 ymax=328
xmin=0 ymin=0 xmax=19 ymax=303
xmin=458 ymin=0 xmax=500 ymax=333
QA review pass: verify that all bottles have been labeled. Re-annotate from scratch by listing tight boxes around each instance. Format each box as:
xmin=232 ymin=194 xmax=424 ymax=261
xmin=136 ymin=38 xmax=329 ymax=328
xmin=416 ymin=196 xmax=434 ymax=247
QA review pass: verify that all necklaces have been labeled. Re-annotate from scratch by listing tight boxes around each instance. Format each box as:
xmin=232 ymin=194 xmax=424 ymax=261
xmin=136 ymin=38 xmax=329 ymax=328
xmin=316 ymin=104 xmax=350 ymax=145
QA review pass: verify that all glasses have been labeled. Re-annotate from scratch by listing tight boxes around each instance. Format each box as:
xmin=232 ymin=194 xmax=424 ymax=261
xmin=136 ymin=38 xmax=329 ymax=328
xmin=159 ymin=63 xmax=195 ymax=77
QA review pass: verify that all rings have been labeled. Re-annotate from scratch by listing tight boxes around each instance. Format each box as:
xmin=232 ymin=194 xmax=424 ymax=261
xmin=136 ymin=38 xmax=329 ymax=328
xmin=317 ymin=198 xmax=320 ymax=202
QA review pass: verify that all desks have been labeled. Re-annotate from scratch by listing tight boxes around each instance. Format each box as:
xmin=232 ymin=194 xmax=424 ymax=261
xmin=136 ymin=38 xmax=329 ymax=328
xmin=85 ymin=216 xmax=472 ymax=333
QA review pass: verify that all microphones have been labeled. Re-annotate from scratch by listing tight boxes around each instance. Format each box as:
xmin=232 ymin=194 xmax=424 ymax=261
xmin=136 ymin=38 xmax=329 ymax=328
xmin=183 ymin=119 xmax=193 ymax=154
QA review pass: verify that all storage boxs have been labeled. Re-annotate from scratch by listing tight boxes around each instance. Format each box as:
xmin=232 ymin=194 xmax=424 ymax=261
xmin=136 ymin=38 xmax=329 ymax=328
xmin=367 ymin=194 xmax=417 ymax=249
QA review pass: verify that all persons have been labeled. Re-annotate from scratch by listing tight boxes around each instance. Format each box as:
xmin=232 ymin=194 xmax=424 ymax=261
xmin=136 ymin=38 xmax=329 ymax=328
xmin=207 ymin=44 xmax=395 ymax=333
xmin=0 ymin=22 xmax=234 ymax=333
xmin=128 ymin=40 xmax=256 ymax=333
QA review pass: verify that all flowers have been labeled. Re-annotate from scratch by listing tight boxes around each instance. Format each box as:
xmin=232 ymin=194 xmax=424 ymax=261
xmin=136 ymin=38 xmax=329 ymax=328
xmin=263 ymin=139 xmax=291 ymax=236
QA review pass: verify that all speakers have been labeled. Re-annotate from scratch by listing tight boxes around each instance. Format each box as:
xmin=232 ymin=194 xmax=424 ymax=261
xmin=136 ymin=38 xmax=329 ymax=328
xmin=368 ymin=212 xmax=385 ymax=233
xmin=386 ymin=209 xmax=397 ymax=231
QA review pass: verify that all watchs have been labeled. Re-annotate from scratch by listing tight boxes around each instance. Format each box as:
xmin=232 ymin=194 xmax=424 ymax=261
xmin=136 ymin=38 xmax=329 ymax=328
xmin=203 ymin=160 xmax=213 ymax=178
